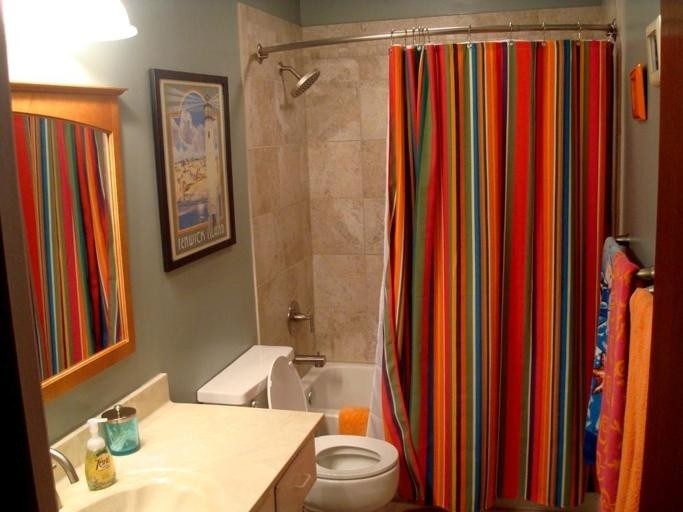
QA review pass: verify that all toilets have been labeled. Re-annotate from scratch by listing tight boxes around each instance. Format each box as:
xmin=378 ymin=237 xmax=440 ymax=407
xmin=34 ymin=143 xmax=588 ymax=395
xmin=196 ymin=344 xmax=401 ymax=512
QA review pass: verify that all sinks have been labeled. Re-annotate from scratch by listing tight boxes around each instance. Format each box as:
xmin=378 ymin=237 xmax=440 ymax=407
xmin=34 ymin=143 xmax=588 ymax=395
xmin=58 ymin=467 xmax=227 ymax=511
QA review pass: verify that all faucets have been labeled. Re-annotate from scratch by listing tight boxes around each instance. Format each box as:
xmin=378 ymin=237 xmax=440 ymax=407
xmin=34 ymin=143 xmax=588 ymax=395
xmin=292 ymin=351 xmax=326 ymax=368
xmin=48 ymin=447 xmax=79 ymax=510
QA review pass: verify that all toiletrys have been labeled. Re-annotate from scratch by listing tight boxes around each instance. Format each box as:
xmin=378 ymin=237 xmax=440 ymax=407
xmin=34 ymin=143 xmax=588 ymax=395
xmin=84 ymin=418 xmax=120 ymax=491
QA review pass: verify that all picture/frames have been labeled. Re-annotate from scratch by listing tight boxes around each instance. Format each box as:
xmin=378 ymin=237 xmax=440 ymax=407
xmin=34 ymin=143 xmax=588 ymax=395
xmin=148 ymin=65 xmax=239 ymax=274
xmin=627 ymin=15 xmax=664 ymax=123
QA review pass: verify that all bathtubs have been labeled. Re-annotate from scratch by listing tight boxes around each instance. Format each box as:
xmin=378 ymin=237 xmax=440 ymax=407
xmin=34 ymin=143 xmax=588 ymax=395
xmin=301 ymin=361 xmax=375 ymax=436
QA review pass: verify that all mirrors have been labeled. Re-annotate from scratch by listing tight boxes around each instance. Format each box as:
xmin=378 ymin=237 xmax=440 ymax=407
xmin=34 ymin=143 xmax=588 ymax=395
xmin=9 ymin=79 xmax=140 ymax=403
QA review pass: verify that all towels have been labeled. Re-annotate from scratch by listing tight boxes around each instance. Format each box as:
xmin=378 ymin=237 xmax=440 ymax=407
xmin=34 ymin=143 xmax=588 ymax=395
xmin=581 ymin=233 xmax=653 ymax=511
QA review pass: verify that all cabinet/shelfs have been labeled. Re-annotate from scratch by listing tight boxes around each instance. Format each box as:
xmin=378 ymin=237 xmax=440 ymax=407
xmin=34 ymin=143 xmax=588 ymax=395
xmin=248 ymin=433 xmax=320 ymax=512
